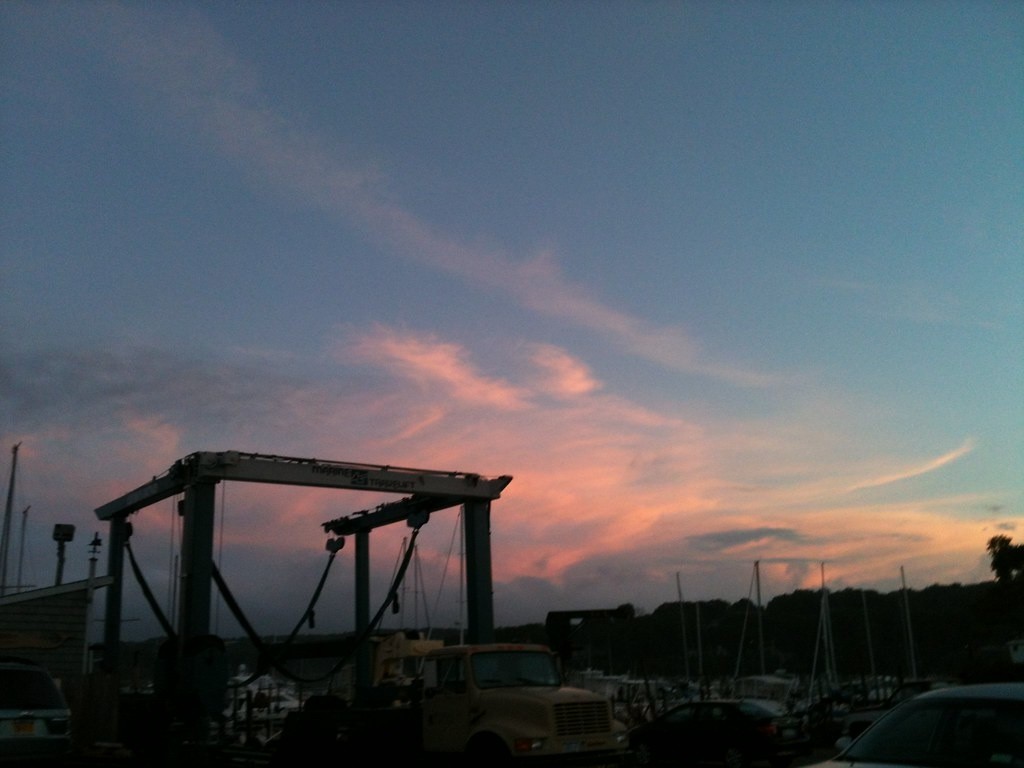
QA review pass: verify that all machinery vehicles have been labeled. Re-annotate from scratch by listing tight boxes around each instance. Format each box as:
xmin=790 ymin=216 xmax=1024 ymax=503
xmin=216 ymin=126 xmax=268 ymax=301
xmin=334 ymin=627 xmax=630 ymax=767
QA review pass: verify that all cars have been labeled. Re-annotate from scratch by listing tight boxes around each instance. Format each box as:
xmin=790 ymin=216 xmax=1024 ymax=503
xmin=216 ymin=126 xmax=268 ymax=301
xmin=0 ymin=655 xmax=72 ymax=764
xmin=803 ymin=682 xmax=1024 ymax=767
xmin=615 ymin=698 xmax=811 ymax=767
